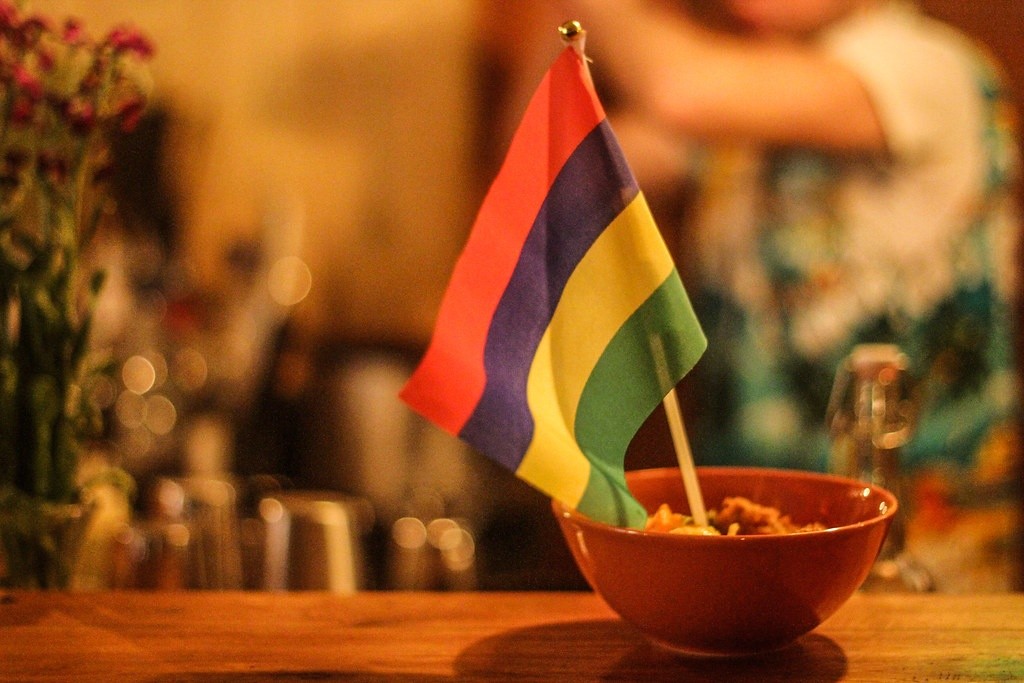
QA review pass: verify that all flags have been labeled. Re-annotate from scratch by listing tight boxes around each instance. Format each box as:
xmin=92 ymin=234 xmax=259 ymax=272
xmin=398 ymin=20 xmax=707 ymax=530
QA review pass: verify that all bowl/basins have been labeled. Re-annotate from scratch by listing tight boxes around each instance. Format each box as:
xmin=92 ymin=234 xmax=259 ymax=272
xmin=551 ymin=466 xmax=898 ymax=658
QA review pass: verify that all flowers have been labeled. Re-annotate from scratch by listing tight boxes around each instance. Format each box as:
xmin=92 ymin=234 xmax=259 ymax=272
xmin=0 ymin=0 xmax=157 ymax=591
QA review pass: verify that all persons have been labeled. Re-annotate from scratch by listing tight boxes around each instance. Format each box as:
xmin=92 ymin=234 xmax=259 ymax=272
xmin=498 ymin=0 xmax=1020 ymax=591
